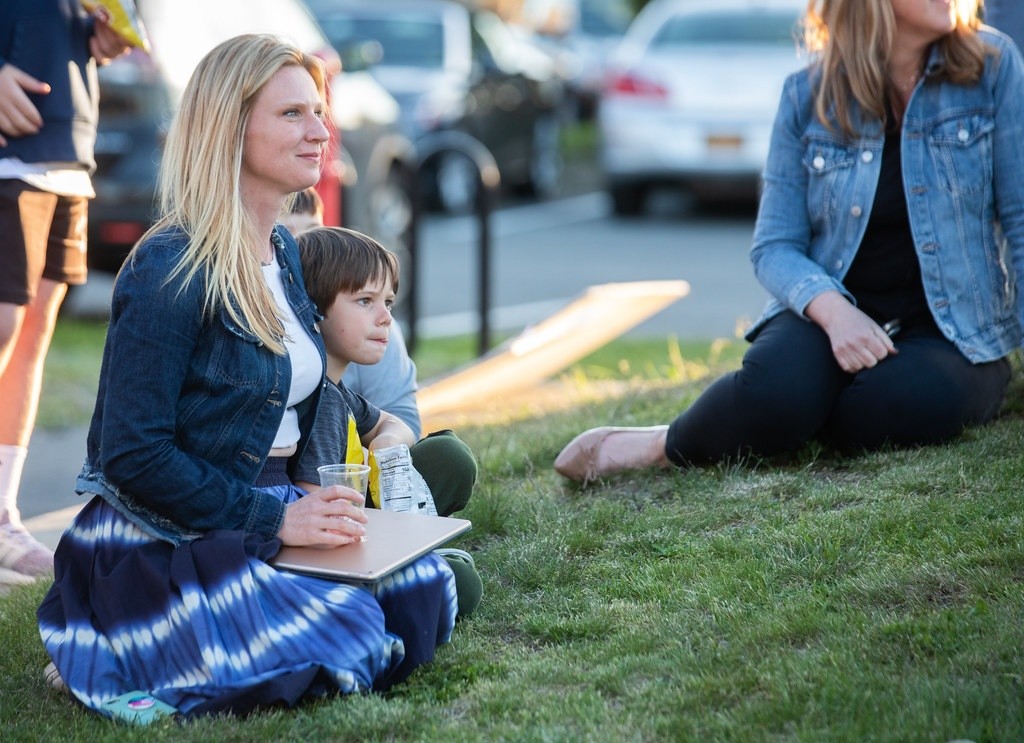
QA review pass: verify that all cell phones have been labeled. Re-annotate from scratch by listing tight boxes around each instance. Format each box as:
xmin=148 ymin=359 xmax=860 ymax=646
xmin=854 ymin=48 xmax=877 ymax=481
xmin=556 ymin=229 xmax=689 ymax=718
xmin=884 ymin=318 xmax=901 ymax=332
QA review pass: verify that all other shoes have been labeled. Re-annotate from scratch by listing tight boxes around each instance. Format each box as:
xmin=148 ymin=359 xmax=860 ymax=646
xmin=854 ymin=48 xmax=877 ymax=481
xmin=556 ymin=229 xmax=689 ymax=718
xmin=552 ymin=424 xmax=674 ymax=481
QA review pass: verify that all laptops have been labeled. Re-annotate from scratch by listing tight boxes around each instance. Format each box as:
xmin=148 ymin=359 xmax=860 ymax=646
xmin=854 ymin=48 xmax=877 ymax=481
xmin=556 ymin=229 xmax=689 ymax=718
xmin=273 ymin=508 xmax=471 ymax=585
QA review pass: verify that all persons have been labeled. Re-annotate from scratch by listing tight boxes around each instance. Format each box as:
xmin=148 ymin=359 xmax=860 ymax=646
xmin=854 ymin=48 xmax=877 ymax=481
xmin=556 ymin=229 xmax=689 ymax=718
xmin=0 ymin=0 xmax=130 ymax=585
xmin=552 ymin=0 xmax=1023 ymax=481
xmin=38 ymin=31 xmax=479 ymax=718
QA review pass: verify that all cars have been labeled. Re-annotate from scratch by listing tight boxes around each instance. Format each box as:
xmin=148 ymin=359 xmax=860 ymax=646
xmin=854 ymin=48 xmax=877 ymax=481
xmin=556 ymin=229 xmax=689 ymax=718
xmin=85 ymin=1 xmax=636 ymax=277
xmin=595 ymin=1 xmax=823 ymax=223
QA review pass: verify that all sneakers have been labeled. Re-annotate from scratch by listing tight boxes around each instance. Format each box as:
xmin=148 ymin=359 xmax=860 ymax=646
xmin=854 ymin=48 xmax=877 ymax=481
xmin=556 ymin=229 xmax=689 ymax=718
xmin=0 ymin=506 xmax=55 ymax=584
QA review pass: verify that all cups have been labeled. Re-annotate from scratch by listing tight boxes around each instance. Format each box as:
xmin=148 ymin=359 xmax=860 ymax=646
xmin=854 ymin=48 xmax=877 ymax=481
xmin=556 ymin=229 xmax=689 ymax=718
xmin=316 ymin=464 xmax=371 ymax=537
